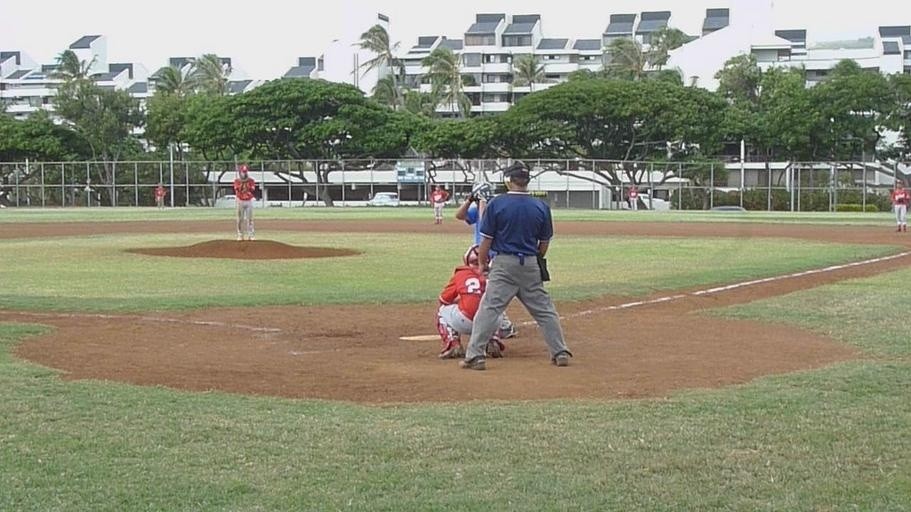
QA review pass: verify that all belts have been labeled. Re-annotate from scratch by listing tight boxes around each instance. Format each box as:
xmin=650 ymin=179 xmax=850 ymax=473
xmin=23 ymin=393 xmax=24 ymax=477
xmin=496 ymin=251 xmax=523 ymax=265
xmin=461 ymin=310 xmax=472 ymax=321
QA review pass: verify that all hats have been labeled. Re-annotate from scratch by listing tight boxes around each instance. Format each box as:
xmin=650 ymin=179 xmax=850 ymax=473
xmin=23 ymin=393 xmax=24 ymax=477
xmin=239 ymin=164 xmax=248 ymax=173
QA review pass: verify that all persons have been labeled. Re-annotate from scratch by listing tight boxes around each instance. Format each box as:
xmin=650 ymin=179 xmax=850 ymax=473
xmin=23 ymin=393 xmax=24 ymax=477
xmin=435 ymin=244 xmax=507 ymax=358
xmin=154 ymin=182 xmax=166 ymax=211
xmin=429 ymin=183 xmax=449 ymax=224
xmin=890 ymin=180 xmax=911 ymax=233
xmin=458 ymin=164 xmax=575 ymax=370
xmin=233 ymin=164 xmax=256 ymax=242
xmin=455 ymin=181 xmax=519 ymax=340
xmin=628 ymin=183 xmax=639 ymax=210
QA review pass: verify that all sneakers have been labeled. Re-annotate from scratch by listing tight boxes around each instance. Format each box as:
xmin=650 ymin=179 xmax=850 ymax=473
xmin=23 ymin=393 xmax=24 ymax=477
xmin=438 ymin=341 xmax=463 ymax=359
xmin=458 ymin=356 xmax=486 ymax=370
xmin=487 ymin=339 xmax=504 ymax=359
xmin=554 ymin=351 xmax=568 ymax=366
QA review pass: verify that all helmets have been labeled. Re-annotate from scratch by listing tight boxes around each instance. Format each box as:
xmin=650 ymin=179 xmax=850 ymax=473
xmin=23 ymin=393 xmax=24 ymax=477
xmin=502 ymin=159 xmax=529 ymax=190
xmin=462 ymin=244 xmax=490 ymax=267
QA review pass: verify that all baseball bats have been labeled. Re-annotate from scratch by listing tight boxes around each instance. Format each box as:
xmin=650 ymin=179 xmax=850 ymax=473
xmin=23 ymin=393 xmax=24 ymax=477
xmin=486 ymin=192 xmax=548 ymax=197
